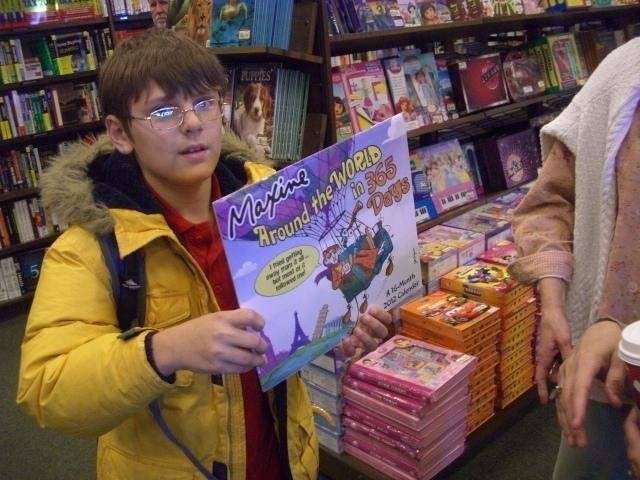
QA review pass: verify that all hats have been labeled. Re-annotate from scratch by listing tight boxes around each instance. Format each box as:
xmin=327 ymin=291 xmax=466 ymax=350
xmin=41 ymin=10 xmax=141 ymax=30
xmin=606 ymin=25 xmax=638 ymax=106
xmin=322 ymin=243 xmax=339 ymax=267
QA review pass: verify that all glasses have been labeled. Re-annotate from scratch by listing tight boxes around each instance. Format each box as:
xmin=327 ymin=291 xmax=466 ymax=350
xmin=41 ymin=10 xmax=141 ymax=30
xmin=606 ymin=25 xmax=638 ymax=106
xmin=127 ymin=94 xmax=229 ymax=133
xmin=326 ymin=249 xmax=337 ymax=263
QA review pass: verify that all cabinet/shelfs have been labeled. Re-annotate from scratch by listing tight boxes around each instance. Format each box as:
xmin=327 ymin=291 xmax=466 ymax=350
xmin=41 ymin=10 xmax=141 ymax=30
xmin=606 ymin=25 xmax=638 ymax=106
xmin=2 ymin=0 xmax=326 ymax=315
xmin=318 ymin=0 xmax=638 ymax=276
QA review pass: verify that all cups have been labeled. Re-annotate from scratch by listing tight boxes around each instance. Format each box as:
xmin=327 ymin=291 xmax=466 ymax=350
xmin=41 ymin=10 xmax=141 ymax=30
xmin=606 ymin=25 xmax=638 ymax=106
xmin=617 ymin=321 xmax=640 ymax=410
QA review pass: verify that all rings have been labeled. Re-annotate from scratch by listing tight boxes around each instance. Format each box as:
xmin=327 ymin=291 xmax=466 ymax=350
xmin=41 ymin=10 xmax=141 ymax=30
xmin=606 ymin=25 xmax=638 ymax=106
xmin=549 ymin=386 xmax=562 ymax=400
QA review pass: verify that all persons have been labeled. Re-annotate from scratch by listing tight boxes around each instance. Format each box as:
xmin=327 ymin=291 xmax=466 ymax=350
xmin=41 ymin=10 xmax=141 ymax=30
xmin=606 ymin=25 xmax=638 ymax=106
xmin=504 ymin=32 xmax=640 ymax=479
xmin=554 ymin=313 xmax=639 ymax=480
xmin=14 ymin=27 xmax=393 ymax=478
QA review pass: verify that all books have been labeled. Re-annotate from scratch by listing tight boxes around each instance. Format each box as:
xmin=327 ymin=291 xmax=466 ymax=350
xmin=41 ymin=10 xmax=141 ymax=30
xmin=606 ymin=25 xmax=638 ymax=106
xmin=213 ymin=108 xmax=426 ymax=394
xmin=0 ymin=1 xmax=311 ymax=307
xmin=295 ymin=222 xmax=547 ymax=479
xmin=328 ymin=1 xmax=639 ymax=228
